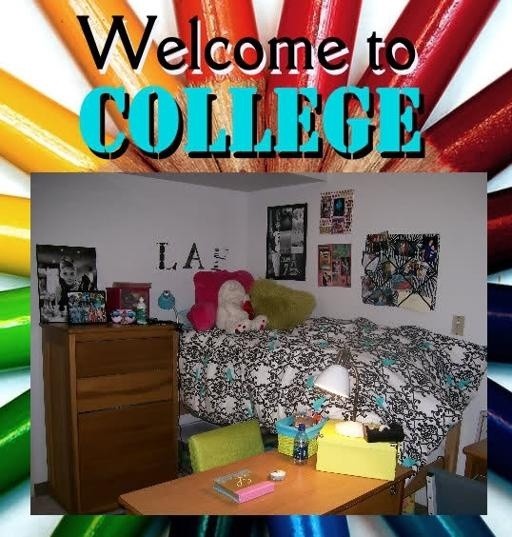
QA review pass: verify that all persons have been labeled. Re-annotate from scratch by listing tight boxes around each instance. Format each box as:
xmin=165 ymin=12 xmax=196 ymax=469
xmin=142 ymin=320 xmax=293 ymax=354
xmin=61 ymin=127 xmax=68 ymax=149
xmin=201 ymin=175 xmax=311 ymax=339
xmin=52 ymin=253 xmax=91 ymax=306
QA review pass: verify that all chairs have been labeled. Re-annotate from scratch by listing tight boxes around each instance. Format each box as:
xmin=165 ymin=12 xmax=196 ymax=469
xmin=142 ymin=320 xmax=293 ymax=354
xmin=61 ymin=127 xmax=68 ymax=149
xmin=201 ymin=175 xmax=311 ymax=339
xmin=184 ymin=417 xmax=263 ymax=474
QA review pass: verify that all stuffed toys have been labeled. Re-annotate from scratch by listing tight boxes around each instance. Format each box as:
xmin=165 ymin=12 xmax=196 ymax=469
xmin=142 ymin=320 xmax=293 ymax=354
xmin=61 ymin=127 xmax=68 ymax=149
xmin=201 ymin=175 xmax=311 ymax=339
xmin=216 ymin=279 xmax=268 ymax=336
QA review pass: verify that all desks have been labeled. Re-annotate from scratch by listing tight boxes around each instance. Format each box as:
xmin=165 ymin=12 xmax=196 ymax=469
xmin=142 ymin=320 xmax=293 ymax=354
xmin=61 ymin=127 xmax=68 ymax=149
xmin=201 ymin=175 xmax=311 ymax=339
xmin=462 ymin=437 xmax=487 ymax=482
xmin=117 ymin=446 xmax=415 ymax=518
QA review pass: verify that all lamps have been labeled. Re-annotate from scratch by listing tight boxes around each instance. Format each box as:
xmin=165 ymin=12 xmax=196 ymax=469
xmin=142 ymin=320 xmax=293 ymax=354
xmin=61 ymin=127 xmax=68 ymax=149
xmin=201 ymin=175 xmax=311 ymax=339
xmin=157 ymin=288 xmax=179 ymax=327
xmin=313 ymin=346 xmax=361 ymax=422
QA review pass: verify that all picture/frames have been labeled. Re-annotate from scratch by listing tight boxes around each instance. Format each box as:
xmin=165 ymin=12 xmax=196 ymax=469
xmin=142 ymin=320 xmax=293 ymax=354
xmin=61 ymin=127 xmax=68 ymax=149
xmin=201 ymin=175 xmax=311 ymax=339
xmin=265 ymin=201 xmax=308 ymax=281
xmin=36 ymin=244 xmax=109 ymax=324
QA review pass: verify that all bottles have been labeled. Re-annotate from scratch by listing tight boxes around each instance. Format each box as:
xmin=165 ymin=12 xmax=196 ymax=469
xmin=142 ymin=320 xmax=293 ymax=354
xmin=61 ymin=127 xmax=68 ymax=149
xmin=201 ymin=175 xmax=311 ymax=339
xmin=293 ymin=424 xmax=310 ymax=463
xmin=136 ymin=297 xmax=149 ymax=326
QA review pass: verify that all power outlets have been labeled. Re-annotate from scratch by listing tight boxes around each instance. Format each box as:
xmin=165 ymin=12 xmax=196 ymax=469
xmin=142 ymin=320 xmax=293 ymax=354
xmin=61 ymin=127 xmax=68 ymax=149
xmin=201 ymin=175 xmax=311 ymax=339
xmin=452 ymin=313 xmax=466 ymax=337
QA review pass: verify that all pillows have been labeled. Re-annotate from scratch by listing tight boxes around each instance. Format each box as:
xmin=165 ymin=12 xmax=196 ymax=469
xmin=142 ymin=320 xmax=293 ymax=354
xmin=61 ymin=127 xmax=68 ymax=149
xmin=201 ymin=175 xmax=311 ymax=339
xmin=186 ymin=270 xmax=316 ymax=331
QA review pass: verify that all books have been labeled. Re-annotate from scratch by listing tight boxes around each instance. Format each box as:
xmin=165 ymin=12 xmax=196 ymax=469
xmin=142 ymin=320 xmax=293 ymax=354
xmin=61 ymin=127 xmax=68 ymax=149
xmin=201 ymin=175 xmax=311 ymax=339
xmin=213 ymin=468 xmax=275 ymax=504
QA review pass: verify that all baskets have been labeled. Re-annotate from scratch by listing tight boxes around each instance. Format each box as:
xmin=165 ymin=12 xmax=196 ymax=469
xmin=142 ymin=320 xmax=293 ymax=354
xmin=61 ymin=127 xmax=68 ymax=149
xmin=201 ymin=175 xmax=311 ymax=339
xmin=275 ymin=412 xmax=326 ymax=458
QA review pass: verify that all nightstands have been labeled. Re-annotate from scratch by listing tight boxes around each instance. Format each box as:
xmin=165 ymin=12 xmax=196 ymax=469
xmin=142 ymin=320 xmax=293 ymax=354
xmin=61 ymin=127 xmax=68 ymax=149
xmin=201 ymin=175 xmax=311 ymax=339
xmin=39 ymin=319 xmax=178 ymax=514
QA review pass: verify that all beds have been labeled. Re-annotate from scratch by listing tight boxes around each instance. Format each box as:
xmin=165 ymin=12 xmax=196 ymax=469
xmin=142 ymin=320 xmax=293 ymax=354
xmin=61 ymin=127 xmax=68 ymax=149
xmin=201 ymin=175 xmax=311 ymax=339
xmin=176 ymin=310 xmax=464 ymax=500
